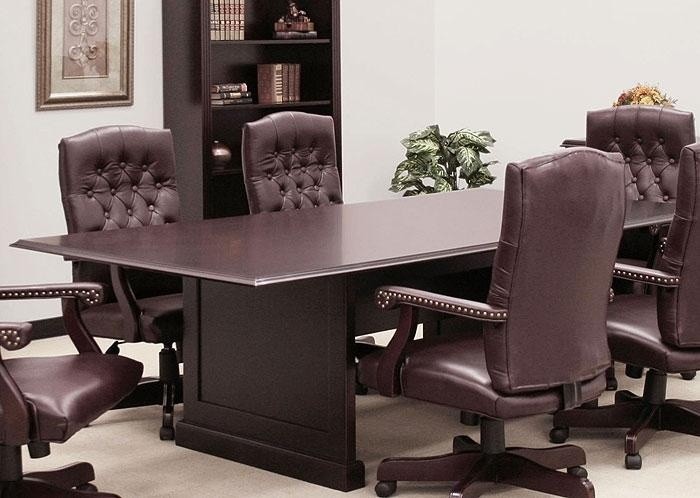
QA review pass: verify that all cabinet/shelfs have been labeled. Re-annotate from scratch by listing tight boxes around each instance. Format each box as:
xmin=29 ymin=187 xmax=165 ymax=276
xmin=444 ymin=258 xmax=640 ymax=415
xmin=162 ymin=0 xmax=342 ymax=222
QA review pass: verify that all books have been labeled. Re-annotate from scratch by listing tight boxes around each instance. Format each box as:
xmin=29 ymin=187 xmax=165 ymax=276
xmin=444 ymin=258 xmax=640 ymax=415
xmin=257 ymin=63 xmax=301 ymax=102
xmin=209 ymin=92 xmax=252 ymax=99
xmin=211 ymin=83 xmax=247 ymax=92
xmin=210 ymin=99 xmax=252 ymax=105
xmin=209 ymin=0 xmax=245 ymax=41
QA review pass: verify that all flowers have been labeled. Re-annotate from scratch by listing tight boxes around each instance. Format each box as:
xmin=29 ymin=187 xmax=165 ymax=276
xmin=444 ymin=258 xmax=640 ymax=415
xmin=618 ymin=84 xmax=677 ymax=107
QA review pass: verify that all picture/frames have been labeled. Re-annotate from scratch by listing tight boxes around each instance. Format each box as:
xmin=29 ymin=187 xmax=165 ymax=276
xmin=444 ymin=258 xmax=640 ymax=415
xmin=35 ymin=0 xmax=134 ymax=111
